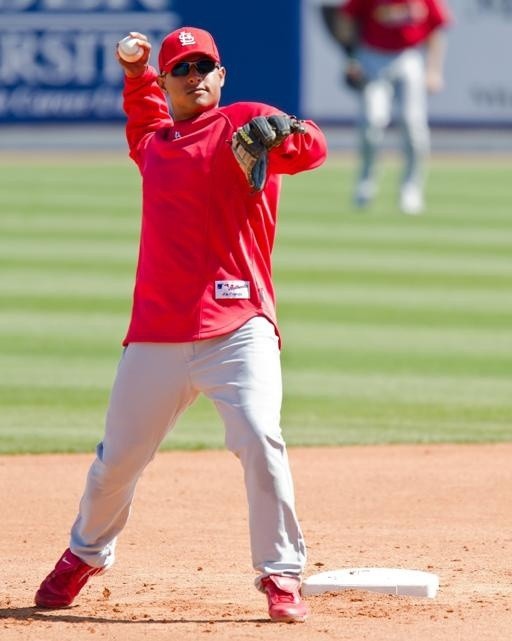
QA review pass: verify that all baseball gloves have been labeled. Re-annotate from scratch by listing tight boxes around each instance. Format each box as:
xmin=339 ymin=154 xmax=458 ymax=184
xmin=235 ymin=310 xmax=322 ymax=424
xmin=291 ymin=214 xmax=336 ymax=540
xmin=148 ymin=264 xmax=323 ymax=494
xmin=226 ymin=115 xmax=308 ymax=193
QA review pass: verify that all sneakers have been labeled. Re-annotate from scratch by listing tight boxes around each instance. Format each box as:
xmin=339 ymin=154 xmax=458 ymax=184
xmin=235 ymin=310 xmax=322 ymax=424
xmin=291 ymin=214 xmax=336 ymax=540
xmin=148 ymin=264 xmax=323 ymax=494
xmin=34 ymin=545 xmax=113 ymax=609
xmin=259 ymin=572 xmax=309 ymax=624
xmin=398 ymin=180 xmax=425 ymax=215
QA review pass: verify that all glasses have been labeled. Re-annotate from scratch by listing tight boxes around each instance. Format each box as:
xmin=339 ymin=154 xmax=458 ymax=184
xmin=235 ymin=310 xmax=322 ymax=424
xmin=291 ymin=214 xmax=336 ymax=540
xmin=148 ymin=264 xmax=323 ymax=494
xmin=167 ymin=59 xmax=221 ymax=77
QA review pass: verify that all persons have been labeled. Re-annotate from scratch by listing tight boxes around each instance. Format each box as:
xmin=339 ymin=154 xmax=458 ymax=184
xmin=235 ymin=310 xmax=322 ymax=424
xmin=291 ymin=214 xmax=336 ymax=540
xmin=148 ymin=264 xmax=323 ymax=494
xmin=30 ymin=27 xmax=332 ymax=625
xmin=326 ymin=1 xmax=455 ymax=214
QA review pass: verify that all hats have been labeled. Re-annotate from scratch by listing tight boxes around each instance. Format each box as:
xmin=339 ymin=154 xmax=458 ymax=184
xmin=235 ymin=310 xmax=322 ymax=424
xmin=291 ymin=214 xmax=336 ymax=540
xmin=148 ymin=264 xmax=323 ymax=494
xmin=158 ymin=26 xmax=221 ymax=75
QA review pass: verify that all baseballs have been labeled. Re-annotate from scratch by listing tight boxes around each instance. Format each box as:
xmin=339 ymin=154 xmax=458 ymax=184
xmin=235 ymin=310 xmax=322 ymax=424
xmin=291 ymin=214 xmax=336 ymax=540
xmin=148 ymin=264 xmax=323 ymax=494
xmin=117 ymin=35 xmax=145 ymax=63
